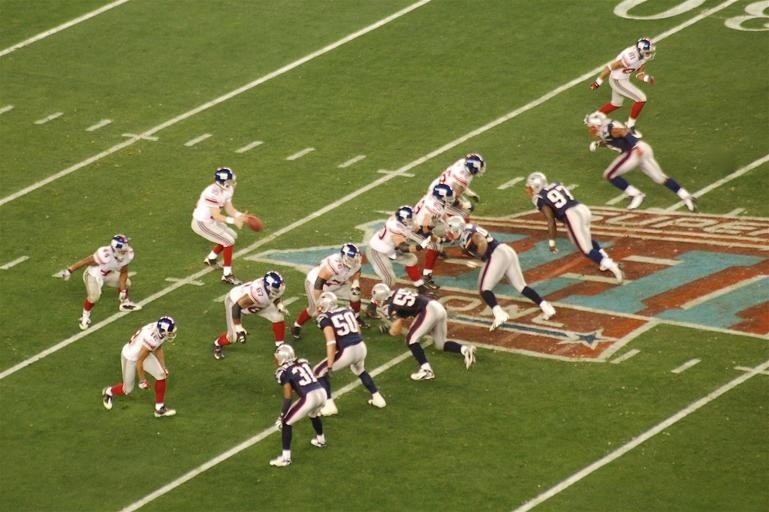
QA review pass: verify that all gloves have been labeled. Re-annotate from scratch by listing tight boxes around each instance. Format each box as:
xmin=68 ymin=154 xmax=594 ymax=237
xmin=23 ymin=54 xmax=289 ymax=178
xmin=472 ymin=194 xmax=480 ymax=203
xmin=589 ymin=140 xmax=600 ymax=153
xmin=138 ymin=379 xmax=150 ymax=391
xmin=235 ymin=323 xmax=247 ymax=343
xmin=278 ymin=302 xmax=291 ymax=323
xmin=324 ymin=366 xmax=332 ymax=377
xmin=53 ymin=268 xmax=70 ymax=281
xmin=548 ymin=246 xmax=560 ymax=254
xmin=590 ymin=78 xmax=603 ymax=90
xmin=350 ymin=287 xmax=360 ymax=298
xmin=462 ymin=202 xmax=474 ymax=213
xmin=119 ymin=290 xmax=126 ymax=301
xmin=276 ymin=415 xmax=283 ymax=429
xmin=379 ymin=323 xmax=390 ymax=334
xmin=644 ymin=74 xmax=655 ymax=85
xmin=421 ymin=236 xmax=433 ymax=249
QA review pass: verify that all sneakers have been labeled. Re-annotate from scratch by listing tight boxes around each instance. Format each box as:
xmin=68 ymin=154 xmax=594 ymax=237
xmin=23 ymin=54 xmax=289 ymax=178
xmin=119 ymin=301 xmax=141 ymax=312
xmin=366 ymin=303 xmax=381 ymax=318
xmin=687 ymin=196 xmax=698 ymax=213
xmin=626 ymin=121 xmax=642 ymax=140
xmin=356 ymin=315 xmax=369 ymax=329
xmin=212 ymin=340 xmax=225 ymax=360
xmin=411 ymin=369 xmax=435 ymax=380
xmin=311 ymin=439 xmax=328 ymax=449
xmin=78 ymin=315 xmax=91 ymax=330
xmin=599 ymin=257 xmax=609 ymax=271
xmin=203 ymin=256 xmax=225 ymax=270
xmin=319 ymin=406 xmax=339 ymax=418
xmin=368 ymin=399 xmax=386 ymax=408
xmin=615 ymin=263 xmax=625 ymax=285
xmin=438 ymin=253 xmax=447 ymax=260
xmin=153 ymin=406 xmax=177 ymax=417
xmin=424 ymin=274 xmax=439 ymax=290
xmin=222 ymin=272 xmax=242 ymax=285
xmin=464 ymin=344 xmax=477 ymax=370
xmin=417 ymin=285 xmax=433 ymax=297
xmin=543 ymin=310 xmax=556 ymax=320
xmin=102 ymin=386 xmax=114 ymax=410
xmin=627 ymin=193 xmax=644 ymax=211
xmin=269 ymin=457 xmax=292 ymax=467
xmin=489 ymin=314 xmax=509 ymax=332
xmin=291 ymin=321 xmax=303 ymax=340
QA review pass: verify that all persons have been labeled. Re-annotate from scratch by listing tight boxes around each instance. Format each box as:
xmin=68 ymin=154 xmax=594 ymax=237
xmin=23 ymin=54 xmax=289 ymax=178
xmin=370 ymin=283 xmax=477 ymax=380
xmin=411 ymin=183 xmax=447 ymax=289
xmin=212 ymin=271 xmax=290 ymax=359
xmin=587 ymin=38 xmax=657 ymax=140
xmin=311 ymin=292 xmax=387 ymax=416
xmin=424 ymin=213 xmax=556 ymax=330
xmin=269 ymin=344 xmax=327 ymax=467
xmin=190 ymin=168 xmax=255 ymax=285
xmin=366 ymin=205 xmax=436 ymax=319
xmin=51 ymin=234 xmax=144 ymax=330
xmin=290 ymin=243 xmax=371 ymax=339
xmin=101 ymin=316 xmax=177 ymax=417
xmin=525 ymin=172 xmax=624 ymax=285
xmin=585 ymin=112 xmax=699 ymax=213
xmin=426 ymin=153 xmax=486 ymax=223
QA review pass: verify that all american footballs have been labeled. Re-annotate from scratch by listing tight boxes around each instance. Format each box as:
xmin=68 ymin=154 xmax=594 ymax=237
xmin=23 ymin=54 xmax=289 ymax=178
xmin=247 ymin=216 xmax=263 ymax=232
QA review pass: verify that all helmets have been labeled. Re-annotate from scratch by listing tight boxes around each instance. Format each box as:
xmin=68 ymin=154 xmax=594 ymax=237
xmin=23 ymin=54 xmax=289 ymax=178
xmin=215 ymin=167 xmax=234 ymax=190
xmin=264 ymin=270 xmax=285 ymax=300
xmin=341 ymin=243 xmax=360 ymax=270
xmin=372 ymin=283 xmax=388 ymax=299
xmin=433 ymin=184 xmax=455 ymax=209
xmin=318 ymin=292 xmax=338 ymax=313
xmin=396 ymin=205 xmax=416 ymax=227
xmin=445 ymin=216 xmax=465 ymax=240
xmin=157 ymin=315 xmax=177 ymax=342
xmin=636 ymin=37 xmax=656 ymax=62
xmin=110 ymin=233 xmax=130 ymax=261
xmin=274 ymin=344 xmax=298 ymax=365
xmin=586 ymin=111 xmax=607 ymax=136
xmin=466 ymin=151 xmax=486 ymax=178
xmin=523 ymin=172 xmax=546 ymax=199
